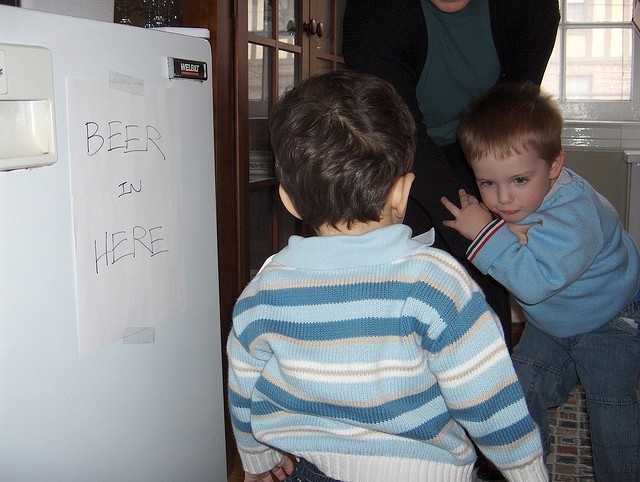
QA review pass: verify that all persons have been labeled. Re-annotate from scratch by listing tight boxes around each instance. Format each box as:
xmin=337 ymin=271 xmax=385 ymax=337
xmin=352 ymin=1 xmax=562 ymax=355
xmin=224 ymin=73 xmax=549 ymax=481
xmin=440 ymin=88 xmax=640 ymax=481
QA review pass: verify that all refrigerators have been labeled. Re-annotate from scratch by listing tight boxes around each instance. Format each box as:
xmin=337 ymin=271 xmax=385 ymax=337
xmin=1 ymin=6 xmax=228 ymax=482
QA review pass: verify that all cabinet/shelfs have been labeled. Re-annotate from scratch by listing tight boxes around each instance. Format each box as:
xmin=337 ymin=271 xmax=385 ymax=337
xmin=113 ymin=0 xmax=344 ymax=479
